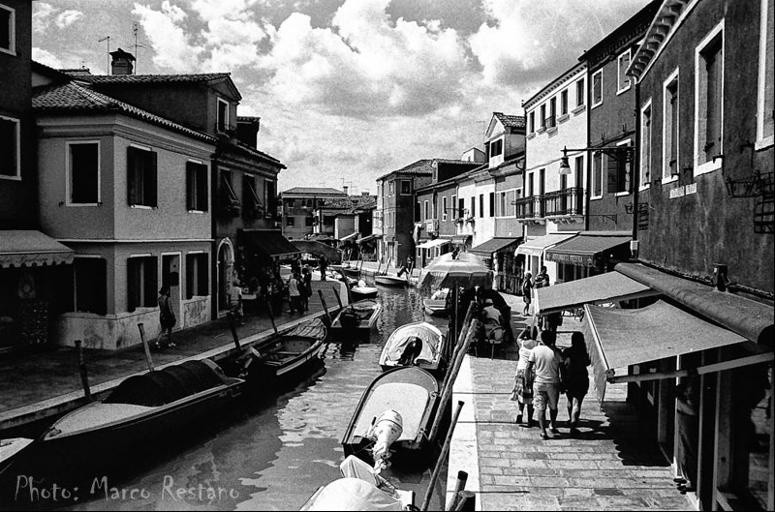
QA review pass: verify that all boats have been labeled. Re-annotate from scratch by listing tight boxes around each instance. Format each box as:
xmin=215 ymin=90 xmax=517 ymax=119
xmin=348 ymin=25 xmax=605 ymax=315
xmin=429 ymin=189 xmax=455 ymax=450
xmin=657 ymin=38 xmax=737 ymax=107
xmin=281 ymin=261 xmax=452 ymax=316
xmin=340 ymin=365 xmax=440 ymax=468
xmin=0 ymin=436 xmax=33 ymax=478
xmin=330 ymin=297 xmax=383 ymax=339
xmin=38 ymin=357 xmax=248 ymax=455
xmin=293 ymin=452 xmax=418 ymax=511
xmin=378 ymin=322 xmax=446 ymax=374
xmin=238 ymin=313 xmax=330 ymax=392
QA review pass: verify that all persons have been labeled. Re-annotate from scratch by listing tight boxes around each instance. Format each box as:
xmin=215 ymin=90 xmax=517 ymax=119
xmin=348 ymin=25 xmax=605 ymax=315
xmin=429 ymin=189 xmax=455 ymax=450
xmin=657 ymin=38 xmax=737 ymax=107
xmin=407 ymin=255 xmax=414 ymax=272
xmin=288 ymin=253 xmax=327 ymax=317
xmin=227 ymin=278 xmax=247 ymax=327
xmin=155 ymin=286 xmax=177 ymax=349
xmin=462 ymin=266 xmax=593 ymax=439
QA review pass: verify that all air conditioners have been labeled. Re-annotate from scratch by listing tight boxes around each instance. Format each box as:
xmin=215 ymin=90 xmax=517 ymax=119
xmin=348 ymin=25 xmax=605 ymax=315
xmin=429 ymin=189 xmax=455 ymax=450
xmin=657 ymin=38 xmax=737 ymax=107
xmin=426 ymin=219 xmax=439 ymax=233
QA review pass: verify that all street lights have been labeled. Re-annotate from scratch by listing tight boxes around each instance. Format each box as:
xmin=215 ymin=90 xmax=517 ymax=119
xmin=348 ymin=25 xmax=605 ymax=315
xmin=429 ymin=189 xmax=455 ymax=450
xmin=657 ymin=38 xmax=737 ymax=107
xmin=366 ymin=216 xmax=385 ymax=263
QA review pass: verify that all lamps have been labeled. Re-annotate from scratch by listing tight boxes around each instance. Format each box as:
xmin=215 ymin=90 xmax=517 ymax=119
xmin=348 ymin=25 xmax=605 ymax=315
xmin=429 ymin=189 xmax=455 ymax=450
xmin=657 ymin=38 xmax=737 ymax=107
xmin=558 ymin=145 xmax=636 ymax=175
